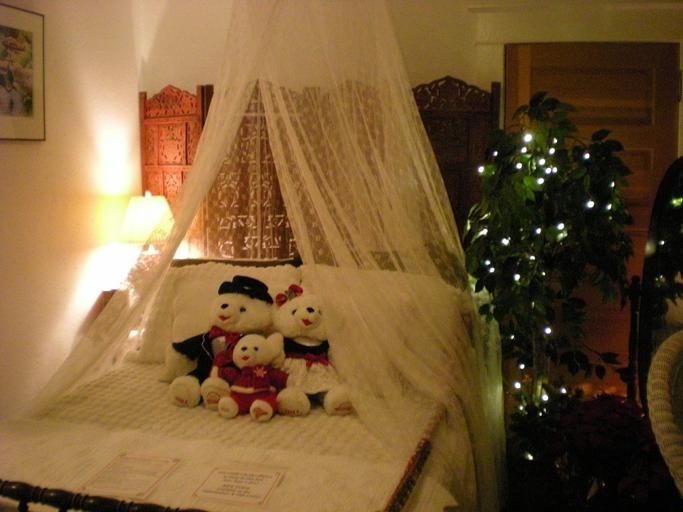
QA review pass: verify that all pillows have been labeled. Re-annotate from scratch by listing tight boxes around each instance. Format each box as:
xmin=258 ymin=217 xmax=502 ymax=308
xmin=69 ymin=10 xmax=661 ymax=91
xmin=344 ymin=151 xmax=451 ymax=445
xmin=160 ymin=278 xmax=408 ymax=393
xmin=131 ymin=263 xmax=301 ymax=362
xmin=294 ymin=264 xmax=460 ymax=395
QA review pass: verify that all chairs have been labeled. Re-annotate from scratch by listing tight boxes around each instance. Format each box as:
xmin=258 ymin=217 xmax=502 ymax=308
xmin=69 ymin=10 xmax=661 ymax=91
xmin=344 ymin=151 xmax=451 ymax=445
xmin=646 ymin=329 xmax=683 ymax=497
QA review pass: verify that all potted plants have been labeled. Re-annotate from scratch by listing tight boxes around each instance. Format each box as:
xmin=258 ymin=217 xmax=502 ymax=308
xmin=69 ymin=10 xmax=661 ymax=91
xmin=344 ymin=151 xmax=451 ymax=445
xmin=461 ymin=90 xmax=635 ymax=510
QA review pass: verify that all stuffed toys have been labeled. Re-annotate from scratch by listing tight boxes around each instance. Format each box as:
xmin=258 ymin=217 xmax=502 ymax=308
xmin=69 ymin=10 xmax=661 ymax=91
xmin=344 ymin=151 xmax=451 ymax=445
xmin=159 ymin=275 xmax=355 ymax=422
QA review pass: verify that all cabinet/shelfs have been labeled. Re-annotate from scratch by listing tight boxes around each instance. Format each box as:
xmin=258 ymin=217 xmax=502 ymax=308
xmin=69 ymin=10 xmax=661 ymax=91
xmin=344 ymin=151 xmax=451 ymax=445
xmin=503 ymin=42 xmax=682 ymax=418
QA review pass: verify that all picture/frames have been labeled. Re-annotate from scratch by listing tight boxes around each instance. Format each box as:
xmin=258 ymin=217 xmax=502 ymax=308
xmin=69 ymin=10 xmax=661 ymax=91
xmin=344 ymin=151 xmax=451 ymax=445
xmin=0 ymin=3 xmax=46 ymax=141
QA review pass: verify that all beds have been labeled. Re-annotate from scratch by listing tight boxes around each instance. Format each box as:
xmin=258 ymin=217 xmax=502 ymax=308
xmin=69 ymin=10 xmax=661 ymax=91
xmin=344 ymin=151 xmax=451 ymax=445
xmin=0 ymin=243 xmax=479 ymax=512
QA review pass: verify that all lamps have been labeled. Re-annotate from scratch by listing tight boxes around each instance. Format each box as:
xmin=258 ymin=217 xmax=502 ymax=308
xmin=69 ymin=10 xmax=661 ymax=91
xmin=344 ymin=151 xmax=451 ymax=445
xmin=122 ymin=191 xmax=178 ymax=256
